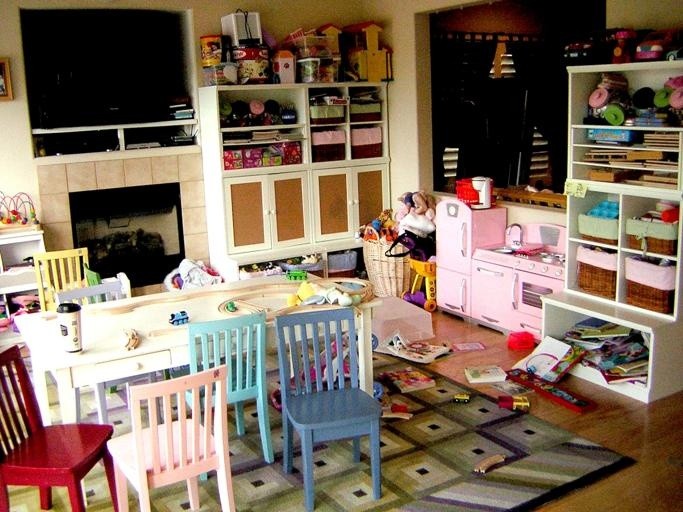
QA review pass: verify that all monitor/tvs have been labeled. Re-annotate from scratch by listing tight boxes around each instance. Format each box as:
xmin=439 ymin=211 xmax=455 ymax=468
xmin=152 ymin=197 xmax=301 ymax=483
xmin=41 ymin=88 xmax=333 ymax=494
xmin=18 ymin=8 xmax=186 ymax=130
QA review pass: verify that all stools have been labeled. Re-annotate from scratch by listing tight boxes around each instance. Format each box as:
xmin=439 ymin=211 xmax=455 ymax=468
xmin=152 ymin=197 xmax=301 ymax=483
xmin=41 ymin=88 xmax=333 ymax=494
xmin=370 ymin=295 xmax=434 ymax=347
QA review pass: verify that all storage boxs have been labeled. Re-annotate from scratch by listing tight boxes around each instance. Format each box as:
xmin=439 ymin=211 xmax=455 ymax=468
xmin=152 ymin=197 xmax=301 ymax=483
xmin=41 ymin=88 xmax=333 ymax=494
xmin=309 ymin=103 xmax=382 ymax=162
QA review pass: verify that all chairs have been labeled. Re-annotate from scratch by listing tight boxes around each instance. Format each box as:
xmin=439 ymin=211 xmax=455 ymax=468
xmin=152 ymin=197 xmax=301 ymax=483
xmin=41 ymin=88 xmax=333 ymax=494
xmin=55 ymin=281 xmax=155 ymax=425
xmin=0 ymin=345 xmax=118 ymax=512
xmin=106 ymin=364 xmax=235 ymax=512
xmin=274 ymin=307 xmax=383 ymax=512
xmin=33 ymin=247 xmax=89 ymax=311
xmin=171 ymin=311 xmax=274 ymax=481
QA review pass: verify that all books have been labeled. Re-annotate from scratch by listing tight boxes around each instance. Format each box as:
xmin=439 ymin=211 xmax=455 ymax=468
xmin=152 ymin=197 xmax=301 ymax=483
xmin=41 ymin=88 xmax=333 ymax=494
xmin=384 ymin=317 xmax=648 ymax=393
xmin=170 ymin=134 xmax=195 ymax=146
xmin=223 ymin=130 xmax=307 ymax=145
xmin=169 ymin=96 xmax=194 ymax=119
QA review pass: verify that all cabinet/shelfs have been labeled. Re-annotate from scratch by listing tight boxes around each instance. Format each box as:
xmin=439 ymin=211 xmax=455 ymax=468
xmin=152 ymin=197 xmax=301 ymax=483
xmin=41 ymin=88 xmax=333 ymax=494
xmin=435 ymin=198 xmax=507 ymax=322
xmin=198 ymin=82 xmax=391 ymax=282
xmin=539 ymin=60 xmax=683 ymax=404
xmin=470 ymin=259 xmax=513 ymax=336
xmin=0 ymin=230 xmax=46 ymax=319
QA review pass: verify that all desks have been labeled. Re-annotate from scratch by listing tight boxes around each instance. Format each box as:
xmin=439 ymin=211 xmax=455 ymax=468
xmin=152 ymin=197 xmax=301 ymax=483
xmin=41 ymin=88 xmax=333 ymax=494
xmin=14 ymin=273 xmax=383 ymax=429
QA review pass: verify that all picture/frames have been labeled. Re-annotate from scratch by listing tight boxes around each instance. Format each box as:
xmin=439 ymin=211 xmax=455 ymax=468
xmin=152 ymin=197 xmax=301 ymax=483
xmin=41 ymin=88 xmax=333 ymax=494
xmin=0 ymin=57 xmax=13 ymax=101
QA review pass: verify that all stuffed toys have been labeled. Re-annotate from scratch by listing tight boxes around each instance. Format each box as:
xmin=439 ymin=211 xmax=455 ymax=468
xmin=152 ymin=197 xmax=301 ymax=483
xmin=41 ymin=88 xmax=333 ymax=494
xmin=359 ymin=191 xmax=436 ymax=262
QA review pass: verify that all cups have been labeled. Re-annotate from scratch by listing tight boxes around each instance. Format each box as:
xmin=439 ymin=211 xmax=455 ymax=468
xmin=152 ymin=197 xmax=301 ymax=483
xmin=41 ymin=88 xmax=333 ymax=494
xmin=57 ymin=303 xmax=83 ymax=353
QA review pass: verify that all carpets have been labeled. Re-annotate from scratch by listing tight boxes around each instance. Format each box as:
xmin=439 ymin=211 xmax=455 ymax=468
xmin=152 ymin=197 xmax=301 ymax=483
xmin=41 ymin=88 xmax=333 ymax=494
xmin=0 ymin=336 xmax=638 ymax=512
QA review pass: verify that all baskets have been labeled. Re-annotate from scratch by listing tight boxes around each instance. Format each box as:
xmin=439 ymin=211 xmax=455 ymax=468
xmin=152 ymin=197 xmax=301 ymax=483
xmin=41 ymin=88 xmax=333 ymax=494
xmin=362 ymin=238 xmax=411 ymax=297
